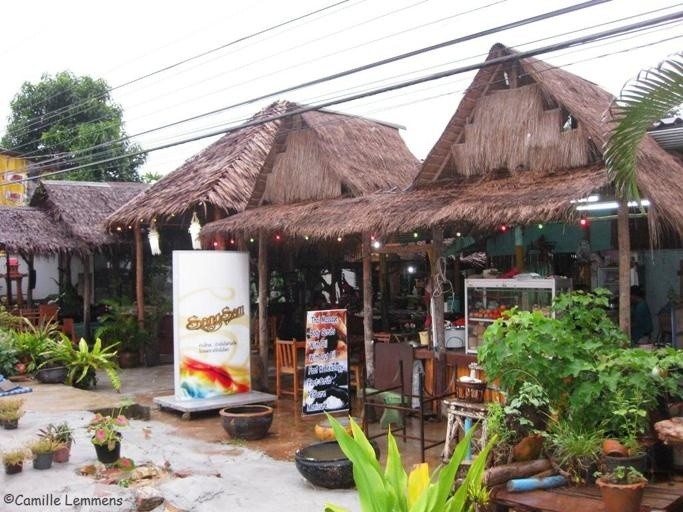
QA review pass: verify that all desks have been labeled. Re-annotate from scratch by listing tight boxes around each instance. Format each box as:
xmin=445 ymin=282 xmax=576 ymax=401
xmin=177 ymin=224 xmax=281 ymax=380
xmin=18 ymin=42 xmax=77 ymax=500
xmin=415 ymin=346 xmax=509 ymax=416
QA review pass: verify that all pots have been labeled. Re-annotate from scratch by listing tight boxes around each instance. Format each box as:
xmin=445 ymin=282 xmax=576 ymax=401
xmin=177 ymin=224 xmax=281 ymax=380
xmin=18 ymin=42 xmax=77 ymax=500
xmin=443 ymin=326 xmax=466 ymax=350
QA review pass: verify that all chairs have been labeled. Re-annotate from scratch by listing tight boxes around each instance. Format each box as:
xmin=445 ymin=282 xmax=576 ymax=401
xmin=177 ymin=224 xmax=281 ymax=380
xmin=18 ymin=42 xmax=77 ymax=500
xmin=440 ymin=362 xmax=512 ymax=463
xmin=273 ymin=335 xmax=304 ymax=403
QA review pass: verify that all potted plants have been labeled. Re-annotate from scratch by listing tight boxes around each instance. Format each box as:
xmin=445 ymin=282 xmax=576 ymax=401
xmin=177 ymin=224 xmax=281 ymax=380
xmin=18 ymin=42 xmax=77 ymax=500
xmin=0 ymin=396 xmax=26 ymax=429
xmin=477 ymin=291 xmax=682 ymax=511
xmin=0 ymin=277 xmax=153 ymax=392
xmin=3 ymin=420 xmax=74 ymax=475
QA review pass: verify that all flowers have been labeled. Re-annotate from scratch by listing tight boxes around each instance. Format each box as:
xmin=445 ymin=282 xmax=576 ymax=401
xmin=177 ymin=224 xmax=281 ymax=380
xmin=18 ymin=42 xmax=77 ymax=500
xmin=86 ymin=414 xmax=127 ymax=451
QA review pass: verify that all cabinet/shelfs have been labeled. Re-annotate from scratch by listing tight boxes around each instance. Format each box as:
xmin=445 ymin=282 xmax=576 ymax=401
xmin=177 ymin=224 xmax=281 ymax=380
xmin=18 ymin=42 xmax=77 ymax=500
xmin=598 ymin=264 xmax=644 ymax=297
xmin=464 ymin=276 xmax=573 ymax=355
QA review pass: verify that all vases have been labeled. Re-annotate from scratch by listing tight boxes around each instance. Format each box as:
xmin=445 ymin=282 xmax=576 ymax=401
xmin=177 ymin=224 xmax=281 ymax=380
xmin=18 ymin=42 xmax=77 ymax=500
xmin=91 ymin=431 xmax=122 ymax=463
